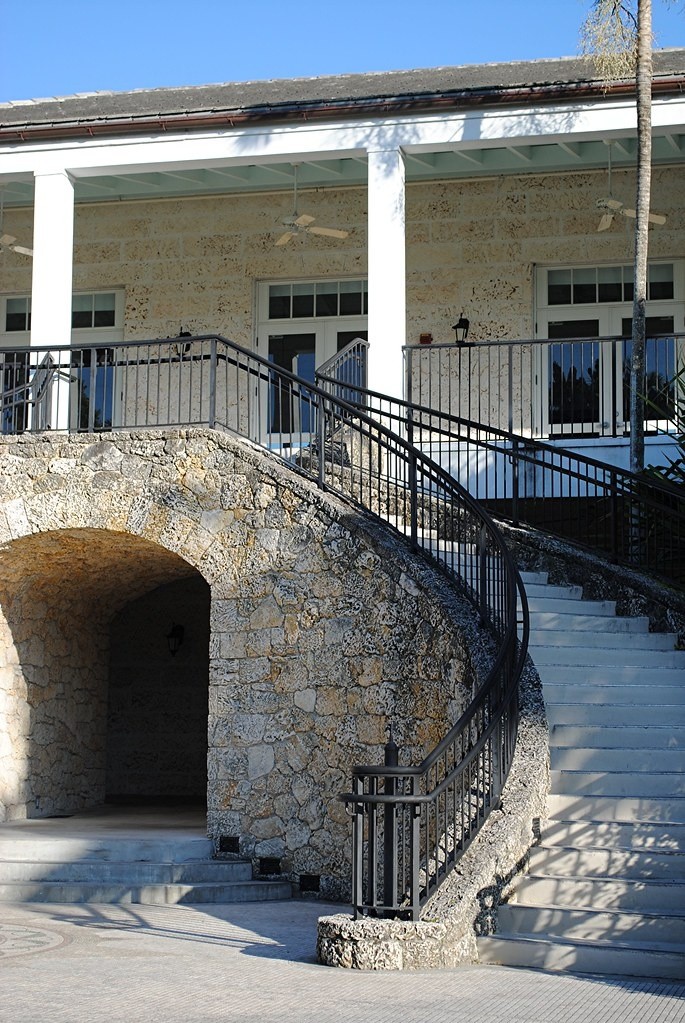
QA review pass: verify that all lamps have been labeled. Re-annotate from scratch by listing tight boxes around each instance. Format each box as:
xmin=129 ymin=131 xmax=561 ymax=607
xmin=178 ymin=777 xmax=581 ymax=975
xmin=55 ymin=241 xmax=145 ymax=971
xmin=175 ymin=325 xmax=193 ymax=356
xmin=451 ymin=311 xmax=469 ymax=345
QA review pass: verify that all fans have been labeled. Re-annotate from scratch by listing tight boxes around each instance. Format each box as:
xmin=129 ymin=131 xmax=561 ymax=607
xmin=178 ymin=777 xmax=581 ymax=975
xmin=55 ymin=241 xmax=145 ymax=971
xmin=590 ymin=138 xmax=667 ymax=235
xmin=273 ymin=161 xmax=348 ymax=247
xmin=0 ymin=183 xmax=33 ymax=257
